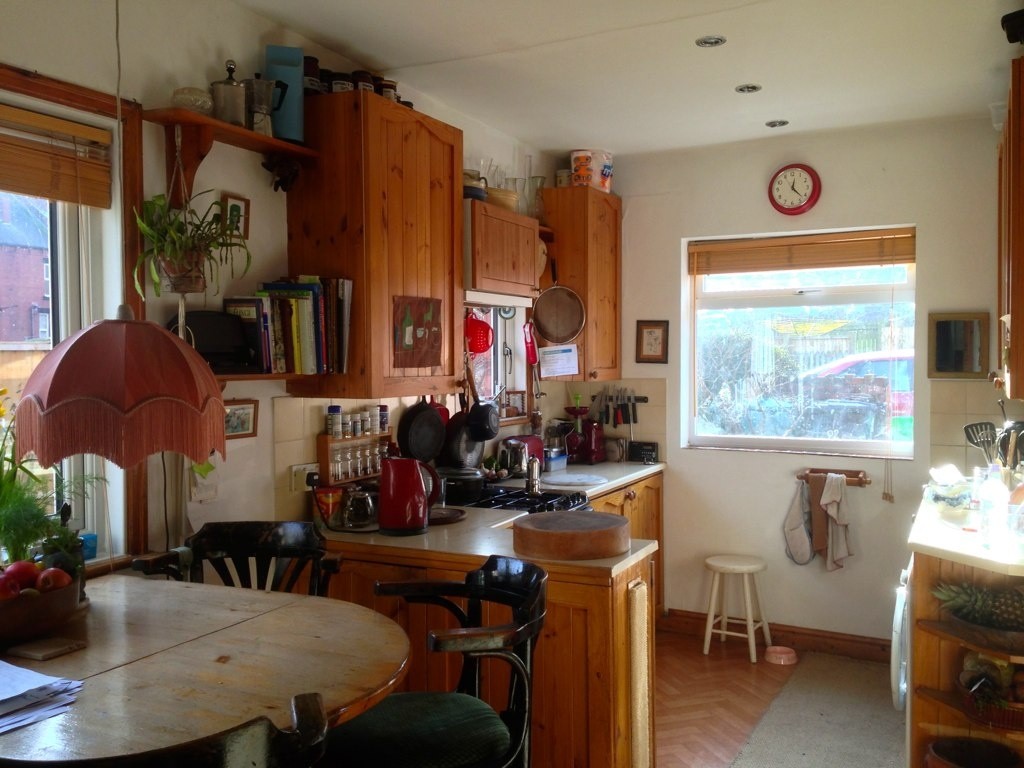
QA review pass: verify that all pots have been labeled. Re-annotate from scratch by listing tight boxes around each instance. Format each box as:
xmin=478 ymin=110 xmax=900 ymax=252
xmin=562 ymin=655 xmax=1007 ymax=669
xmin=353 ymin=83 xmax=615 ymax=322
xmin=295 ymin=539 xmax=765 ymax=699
xmin=396 ymin=363 xmax=500 ymax=505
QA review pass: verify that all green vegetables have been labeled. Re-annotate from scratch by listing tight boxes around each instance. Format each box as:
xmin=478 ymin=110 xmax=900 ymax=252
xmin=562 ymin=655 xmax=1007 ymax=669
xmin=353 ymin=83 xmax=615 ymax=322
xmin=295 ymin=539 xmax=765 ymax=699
xmin=478 ymin=456 xmax=501 ymax=472
xmin=964 ymin=672 xmax=1008 ymax=711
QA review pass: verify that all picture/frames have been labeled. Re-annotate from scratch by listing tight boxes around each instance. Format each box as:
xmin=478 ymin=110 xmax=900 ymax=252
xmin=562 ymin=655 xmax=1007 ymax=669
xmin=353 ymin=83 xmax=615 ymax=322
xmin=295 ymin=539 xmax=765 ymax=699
xmin=221 ymin=193 xmax=250 ymax=239
xmin=636 ymin=320 xmax=669 ymax=364
xmin=223 ymin=400 xmax=258 ymax=440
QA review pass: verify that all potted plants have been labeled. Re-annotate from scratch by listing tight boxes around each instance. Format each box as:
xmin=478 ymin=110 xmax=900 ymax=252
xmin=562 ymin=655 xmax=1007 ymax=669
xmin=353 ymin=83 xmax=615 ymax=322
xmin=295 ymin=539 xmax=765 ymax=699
xmin=132 ymin=188 xmax=252 ymax=297
xmin=0 ymin=383 xmax=110 ymax=575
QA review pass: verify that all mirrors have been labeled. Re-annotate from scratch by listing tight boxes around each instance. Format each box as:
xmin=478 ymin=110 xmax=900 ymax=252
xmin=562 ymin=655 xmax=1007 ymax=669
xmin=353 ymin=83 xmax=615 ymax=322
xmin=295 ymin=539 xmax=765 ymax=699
xmin=928 ymin=312 xmax=989 ymax=379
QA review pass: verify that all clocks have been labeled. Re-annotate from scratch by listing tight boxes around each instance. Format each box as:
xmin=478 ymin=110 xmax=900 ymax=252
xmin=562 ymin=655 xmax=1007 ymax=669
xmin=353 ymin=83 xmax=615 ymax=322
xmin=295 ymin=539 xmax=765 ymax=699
xmin=768 ymin=163 xmax=821 ymax=215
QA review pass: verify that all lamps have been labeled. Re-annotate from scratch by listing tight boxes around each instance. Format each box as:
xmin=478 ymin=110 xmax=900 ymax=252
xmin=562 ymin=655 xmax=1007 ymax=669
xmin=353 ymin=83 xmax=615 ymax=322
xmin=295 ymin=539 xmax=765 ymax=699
xmin=15 ymin=0 xmax=226 ymax=469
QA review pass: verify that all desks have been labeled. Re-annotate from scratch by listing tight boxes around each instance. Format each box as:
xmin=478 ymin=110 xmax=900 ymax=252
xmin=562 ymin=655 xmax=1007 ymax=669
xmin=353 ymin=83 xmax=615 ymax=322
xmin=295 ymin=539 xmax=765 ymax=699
xmin=0 ymin=575 xmax=412 ymax=768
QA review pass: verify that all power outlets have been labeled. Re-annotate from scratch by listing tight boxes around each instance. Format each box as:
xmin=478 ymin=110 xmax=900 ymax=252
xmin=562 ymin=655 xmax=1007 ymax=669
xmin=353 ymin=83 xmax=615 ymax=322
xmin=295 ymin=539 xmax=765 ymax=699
xmin=288 ymin=462 xmax=322 ymax=492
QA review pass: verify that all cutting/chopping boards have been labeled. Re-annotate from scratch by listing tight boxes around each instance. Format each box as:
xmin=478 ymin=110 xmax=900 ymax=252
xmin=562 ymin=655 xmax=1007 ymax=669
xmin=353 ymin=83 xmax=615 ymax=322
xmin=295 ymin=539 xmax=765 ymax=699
xmin=514 ymin=511 xmax=632 ymax=558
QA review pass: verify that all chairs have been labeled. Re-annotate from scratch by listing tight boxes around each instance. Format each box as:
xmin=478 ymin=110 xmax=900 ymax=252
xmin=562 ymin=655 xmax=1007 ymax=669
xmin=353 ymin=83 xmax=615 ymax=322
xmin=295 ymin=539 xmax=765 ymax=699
xmin=320 ymin=555 xmax=547 ymax=768
xmin=131 ymin=522 xmax=340 ymax=598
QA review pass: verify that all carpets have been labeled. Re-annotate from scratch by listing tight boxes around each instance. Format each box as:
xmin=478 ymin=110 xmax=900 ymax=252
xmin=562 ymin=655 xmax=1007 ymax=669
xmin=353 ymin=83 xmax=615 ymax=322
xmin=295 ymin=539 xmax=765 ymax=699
xmin=729 ymin=651 xmax=907 ymax=768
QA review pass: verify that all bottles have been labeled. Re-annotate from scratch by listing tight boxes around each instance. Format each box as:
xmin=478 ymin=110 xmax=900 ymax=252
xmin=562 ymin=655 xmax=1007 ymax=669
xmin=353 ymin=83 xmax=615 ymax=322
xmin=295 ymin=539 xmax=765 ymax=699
xmin=312 ymin=487 xmax=343 ymax=530
xmin=326 ymin=404 xmax=390 ymax=482
xmin=210 ymin=59 xmax=248 ymax=127
xmin=170 ymin=86 xmax=215 ymax=116
xmin=555 ymin=169 xmax=571 ymax=188
xmin=473 ymin=153 xmax=546 ymax=228
xmin=978 ymin=463 xmax=1010 ymax=550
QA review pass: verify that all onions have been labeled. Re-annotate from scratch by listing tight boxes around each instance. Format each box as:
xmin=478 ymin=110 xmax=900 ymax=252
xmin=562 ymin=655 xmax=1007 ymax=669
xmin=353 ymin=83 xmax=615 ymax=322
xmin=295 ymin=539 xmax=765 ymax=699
xmin=481 ymin=464 xmax=508 ymax=479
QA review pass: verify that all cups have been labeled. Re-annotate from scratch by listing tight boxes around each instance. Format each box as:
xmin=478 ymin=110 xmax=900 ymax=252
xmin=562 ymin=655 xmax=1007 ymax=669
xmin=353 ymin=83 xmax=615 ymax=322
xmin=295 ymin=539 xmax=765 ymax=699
xmin=463 ymin=168 xmax=488 ymax=191
xmin=79 ymin=534 xmax=97 ymax=559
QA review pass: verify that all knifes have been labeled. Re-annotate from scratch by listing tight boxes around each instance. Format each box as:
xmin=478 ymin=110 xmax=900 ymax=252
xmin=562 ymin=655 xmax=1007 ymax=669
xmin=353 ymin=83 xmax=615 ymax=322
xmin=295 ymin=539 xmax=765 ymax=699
xmin=598 ymin=384 xmax=637 ymax=428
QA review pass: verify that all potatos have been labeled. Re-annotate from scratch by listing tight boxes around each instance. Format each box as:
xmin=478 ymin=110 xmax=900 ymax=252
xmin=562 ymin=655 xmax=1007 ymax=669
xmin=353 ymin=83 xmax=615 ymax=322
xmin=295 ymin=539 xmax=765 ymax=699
xmin=959 ymin=668 xmax=1024 ymax=704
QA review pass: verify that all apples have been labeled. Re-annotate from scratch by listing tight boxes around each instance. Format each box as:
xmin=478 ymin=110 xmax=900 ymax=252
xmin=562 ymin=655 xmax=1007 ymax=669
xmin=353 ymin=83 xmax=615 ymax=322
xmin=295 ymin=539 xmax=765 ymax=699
xmin=0 ymin=560 xmax=72 ymax=601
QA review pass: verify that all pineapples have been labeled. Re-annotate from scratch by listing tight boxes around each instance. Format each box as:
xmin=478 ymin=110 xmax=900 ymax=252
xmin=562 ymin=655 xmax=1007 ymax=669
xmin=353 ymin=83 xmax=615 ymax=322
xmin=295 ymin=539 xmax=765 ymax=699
xmin=930 ymin=580 xmax=1024 ymax=631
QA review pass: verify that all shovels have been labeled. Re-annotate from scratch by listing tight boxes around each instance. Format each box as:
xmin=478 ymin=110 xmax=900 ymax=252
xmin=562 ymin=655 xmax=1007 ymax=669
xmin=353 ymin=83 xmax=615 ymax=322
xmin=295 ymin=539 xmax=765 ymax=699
xmin=962 ymin=421 xmax=997 ymax=464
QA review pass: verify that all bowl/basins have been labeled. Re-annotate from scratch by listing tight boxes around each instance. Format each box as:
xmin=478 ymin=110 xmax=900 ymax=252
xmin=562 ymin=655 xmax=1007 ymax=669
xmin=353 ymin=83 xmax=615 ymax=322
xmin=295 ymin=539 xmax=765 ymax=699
xmin=545 ymin=454 xmax=568 ymax=471
xmin=485 ymin=187 xmax=520 ymax=214
xmin=0 ymin=567 xmax=80 ymax=646
xmin=462 ymin=186 xmax=487 ymax=201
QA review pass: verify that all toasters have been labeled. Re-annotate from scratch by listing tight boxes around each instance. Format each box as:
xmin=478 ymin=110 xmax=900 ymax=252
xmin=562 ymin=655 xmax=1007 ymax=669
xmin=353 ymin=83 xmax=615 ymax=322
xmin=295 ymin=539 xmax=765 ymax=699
xmin=497 ymin=434 xmax=544 ymax=478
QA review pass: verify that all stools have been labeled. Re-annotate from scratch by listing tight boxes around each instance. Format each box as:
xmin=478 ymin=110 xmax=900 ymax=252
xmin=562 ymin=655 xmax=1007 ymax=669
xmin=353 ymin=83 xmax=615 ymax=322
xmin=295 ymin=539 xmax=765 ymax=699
xmin=703 ymin=555 xmax=772 ymax=663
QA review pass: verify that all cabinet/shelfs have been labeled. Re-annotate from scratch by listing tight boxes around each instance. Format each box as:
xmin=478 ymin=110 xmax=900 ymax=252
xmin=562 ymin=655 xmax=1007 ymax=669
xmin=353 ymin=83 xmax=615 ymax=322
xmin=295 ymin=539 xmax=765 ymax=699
xmin=317 ymin=432 xmax=392 ymax=486
xmin=990 ymin=57 xmax=1024 ymax=400
xmin=142 ymin=89 xmax=621 ymax=398
xmin=907 ymin=477 xmax=1024 ymax=768
xmin=588 ymin=472 xmax=664 ymax=622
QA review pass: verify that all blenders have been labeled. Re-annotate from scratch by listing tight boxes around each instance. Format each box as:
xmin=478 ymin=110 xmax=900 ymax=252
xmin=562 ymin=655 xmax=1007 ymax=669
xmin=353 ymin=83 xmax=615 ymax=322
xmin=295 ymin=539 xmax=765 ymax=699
xmin=240 ymin=72 xmax=288 ymax=137
xmin=556 ymin=406 xmax=606 ymax=465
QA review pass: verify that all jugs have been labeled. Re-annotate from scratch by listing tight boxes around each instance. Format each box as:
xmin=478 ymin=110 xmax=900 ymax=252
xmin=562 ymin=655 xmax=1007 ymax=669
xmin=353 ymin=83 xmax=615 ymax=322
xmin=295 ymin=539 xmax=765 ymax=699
xmin=379 ymin=457 xmax=441 ymax=537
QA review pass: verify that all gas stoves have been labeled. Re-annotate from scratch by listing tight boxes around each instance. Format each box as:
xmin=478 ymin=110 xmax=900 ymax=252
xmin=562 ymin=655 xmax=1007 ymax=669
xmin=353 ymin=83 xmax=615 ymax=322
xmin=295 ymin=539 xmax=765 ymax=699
xmin=461 ymin=486 xmax=595 ymax=513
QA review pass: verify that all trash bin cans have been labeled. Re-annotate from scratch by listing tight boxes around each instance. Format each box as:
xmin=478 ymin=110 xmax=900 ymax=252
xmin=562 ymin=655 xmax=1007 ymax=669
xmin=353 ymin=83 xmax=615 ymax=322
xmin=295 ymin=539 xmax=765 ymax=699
xmin=927 ymin=738 xmax=1024 ymax=768
